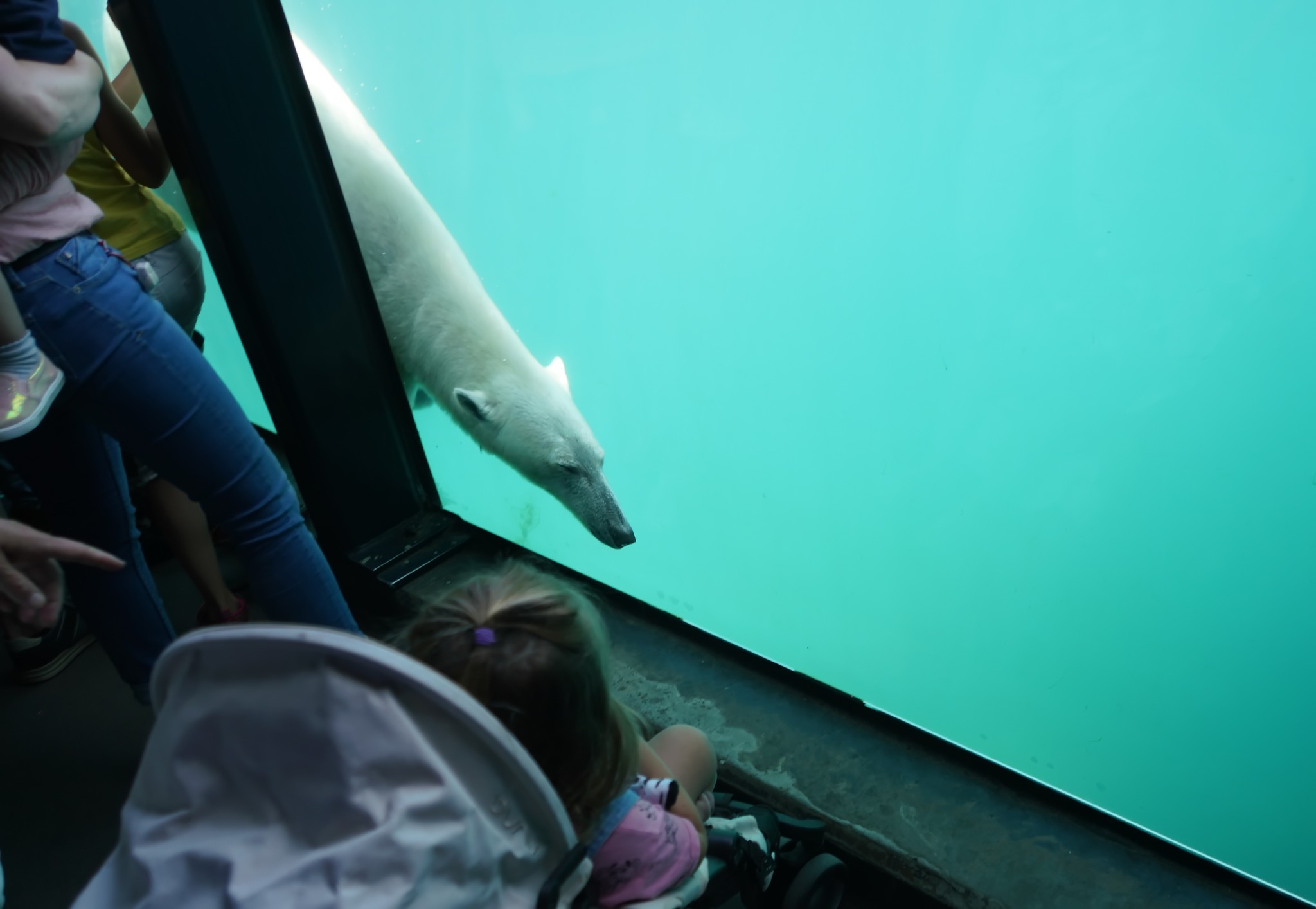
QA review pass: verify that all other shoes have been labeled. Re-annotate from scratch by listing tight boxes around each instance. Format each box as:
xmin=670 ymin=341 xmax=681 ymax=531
xmin=197 ymin=596 xmax=247 ymax=625
xmin=5 ymin=602 xmax=93 ymax=684
xmin=1 ymin=352 xmax=65 ymax=439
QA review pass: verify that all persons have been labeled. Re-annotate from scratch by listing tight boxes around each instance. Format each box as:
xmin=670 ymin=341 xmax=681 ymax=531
xmin=0 ymin=0 xmax=368 ymax=708
xmin=391 ymin=561 xmax=717 ymax=909
